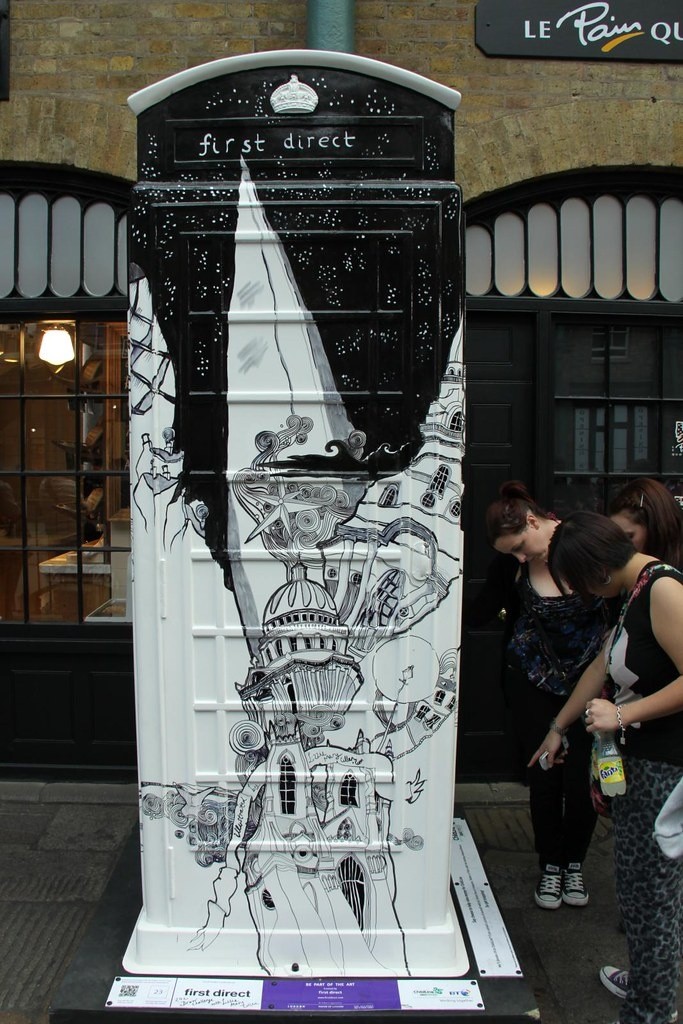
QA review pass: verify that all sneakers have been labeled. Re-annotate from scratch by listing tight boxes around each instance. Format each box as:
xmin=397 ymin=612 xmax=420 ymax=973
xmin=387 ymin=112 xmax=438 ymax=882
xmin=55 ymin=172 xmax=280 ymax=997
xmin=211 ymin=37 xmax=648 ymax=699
xmin=562 ymin=861 xmax=589 ymax=905
xmin=534 ymin=863 xmax=562 ymax=909
xmin=599 ymin=965 xmax=678 ymax=1023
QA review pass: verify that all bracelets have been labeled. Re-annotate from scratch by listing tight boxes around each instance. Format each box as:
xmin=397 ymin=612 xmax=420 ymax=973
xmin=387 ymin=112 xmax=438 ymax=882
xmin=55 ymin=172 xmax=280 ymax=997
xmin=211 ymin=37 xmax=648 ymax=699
xmin=616 ymin=705 xmax=626 ymax=744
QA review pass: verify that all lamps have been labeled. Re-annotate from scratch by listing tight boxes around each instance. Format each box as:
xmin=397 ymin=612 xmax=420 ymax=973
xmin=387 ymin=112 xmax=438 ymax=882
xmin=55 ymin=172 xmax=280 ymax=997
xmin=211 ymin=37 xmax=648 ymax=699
xmin=38 ymin=322 xmax=74 ymax=365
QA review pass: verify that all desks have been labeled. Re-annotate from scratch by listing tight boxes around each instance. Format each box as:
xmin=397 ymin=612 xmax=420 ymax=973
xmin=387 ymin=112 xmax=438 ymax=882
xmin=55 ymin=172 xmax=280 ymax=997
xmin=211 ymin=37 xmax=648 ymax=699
xmin=38 ymin=538 xmax=112 ymax=622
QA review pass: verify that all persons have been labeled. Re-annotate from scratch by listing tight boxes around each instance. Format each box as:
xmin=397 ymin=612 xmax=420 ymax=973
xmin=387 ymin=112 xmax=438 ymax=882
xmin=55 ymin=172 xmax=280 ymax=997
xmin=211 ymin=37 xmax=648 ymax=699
xmin=478 ymin=481 xmax=602 ymax=909
xmin=524 ymin=510 xmax=683 ymax=1024
xmin=0 ymin=448 xmax=132 ymax=623
xmin=590 ymin=477 xmax=682 ymax=1005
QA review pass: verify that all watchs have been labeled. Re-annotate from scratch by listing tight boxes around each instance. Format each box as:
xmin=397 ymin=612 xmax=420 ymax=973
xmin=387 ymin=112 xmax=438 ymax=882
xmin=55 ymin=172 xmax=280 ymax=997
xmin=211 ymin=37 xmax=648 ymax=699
xmin=550 ymin=720 xmax=569 ymax=736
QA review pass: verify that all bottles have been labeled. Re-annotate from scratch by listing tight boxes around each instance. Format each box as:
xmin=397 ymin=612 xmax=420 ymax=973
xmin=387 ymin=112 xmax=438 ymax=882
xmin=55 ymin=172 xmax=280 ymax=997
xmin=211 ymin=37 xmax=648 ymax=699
xmin=590 ymin=730 xmax=632 ymax=820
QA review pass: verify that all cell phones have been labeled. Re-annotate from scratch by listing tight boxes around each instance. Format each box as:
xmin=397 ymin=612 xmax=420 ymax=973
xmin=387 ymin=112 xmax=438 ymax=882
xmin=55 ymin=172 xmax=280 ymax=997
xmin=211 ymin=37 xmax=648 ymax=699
xmin=539 ymin=736 xmax=569 ymax=771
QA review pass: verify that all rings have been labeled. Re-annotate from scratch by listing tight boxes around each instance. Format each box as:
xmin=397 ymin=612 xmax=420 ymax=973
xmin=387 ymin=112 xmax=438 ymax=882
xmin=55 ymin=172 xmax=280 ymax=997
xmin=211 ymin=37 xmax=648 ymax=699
xmin=585 ymin=709 xmax=592 ymax=717
